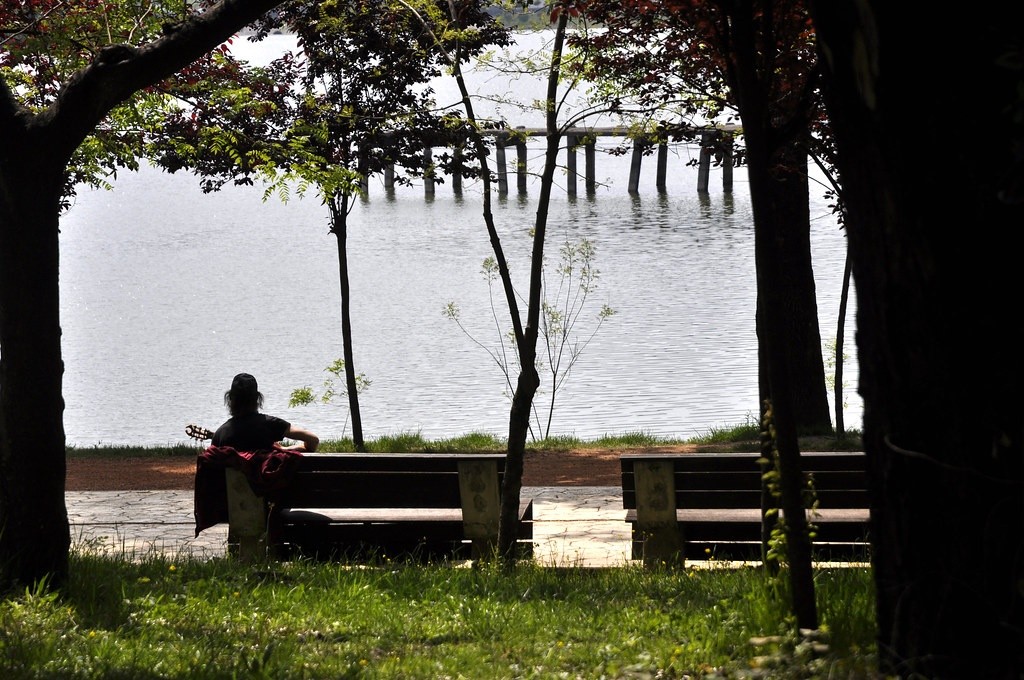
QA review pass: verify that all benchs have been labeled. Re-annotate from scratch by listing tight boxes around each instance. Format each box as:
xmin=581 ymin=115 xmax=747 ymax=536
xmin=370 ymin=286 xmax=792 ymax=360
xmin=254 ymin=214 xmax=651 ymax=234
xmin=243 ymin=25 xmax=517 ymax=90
xmin=226 ymin=453 xmax=533 ymax=579
xmin=620 ymin=451 xmax=869 ymax=576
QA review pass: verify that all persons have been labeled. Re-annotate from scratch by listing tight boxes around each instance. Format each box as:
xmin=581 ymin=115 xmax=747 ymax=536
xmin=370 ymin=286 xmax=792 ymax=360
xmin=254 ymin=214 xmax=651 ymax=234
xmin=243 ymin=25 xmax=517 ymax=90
xmin=212 ymin=373 xmax=320 ymax=514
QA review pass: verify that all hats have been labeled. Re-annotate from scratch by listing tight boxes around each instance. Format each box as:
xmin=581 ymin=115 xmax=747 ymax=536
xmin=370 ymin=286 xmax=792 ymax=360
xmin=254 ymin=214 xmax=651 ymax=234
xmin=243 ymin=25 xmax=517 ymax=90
xmin=228 ymin=373 xmax=260 ymax=401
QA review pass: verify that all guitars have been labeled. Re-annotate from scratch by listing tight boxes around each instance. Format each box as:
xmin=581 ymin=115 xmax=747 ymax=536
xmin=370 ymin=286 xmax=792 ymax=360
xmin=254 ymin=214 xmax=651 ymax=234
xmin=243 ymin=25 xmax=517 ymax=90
xmin=186 ymin=423 xmax=306 ymax=452
